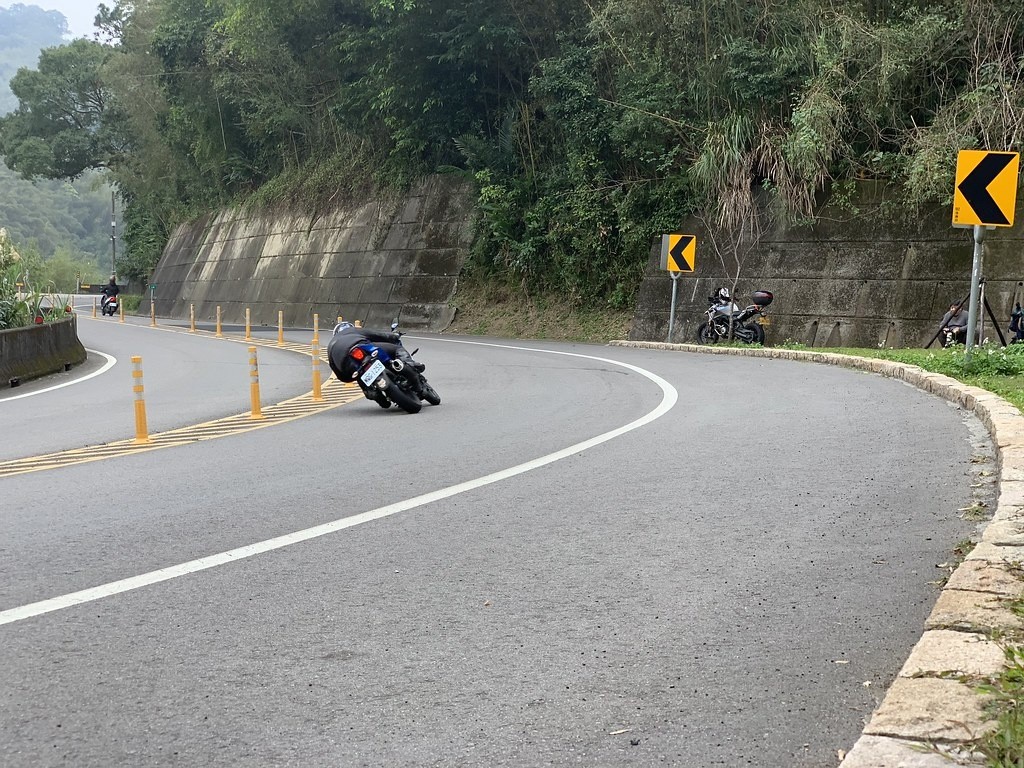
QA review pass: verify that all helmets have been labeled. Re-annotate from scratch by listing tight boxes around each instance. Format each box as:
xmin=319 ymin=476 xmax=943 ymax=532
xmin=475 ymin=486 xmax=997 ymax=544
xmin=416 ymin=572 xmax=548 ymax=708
xmin=714 ymin=287 xmax=730 ymax=304
xmin=109 ymin=276 xmax=115 ymax=281
xmin=333 ymin=321 xmax=354 ymax=337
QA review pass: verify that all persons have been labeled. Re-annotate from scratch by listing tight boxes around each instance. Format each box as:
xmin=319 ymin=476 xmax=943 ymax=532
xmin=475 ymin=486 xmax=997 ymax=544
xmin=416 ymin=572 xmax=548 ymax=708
xmin=327 ymin=321 xmax=426 ymax=409
xmin=938 ymin=300 xmax=977 ymax=348
xmin=100 ymin=276 xmax=120 ymax=298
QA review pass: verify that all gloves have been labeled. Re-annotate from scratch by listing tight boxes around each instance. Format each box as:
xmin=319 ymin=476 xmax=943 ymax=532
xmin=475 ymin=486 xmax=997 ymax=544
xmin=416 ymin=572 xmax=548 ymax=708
xmin=393 ymin=332 xmax=400 ymax=341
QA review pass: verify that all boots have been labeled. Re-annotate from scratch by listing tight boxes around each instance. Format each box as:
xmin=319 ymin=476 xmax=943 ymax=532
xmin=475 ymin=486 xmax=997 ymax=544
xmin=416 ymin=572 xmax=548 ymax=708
xmin=396 ymin=347 xmax=426 ymax=374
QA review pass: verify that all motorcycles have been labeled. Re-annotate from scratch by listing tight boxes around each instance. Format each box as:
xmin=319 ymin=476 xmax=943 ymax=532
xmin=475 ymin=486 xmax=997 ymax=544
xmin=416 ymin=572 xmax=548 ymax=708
xmin=697 ymin=287 xmax=772 ymax=347
xmin=348 ymin=319 xmax=442 ymax=415
xmin=100 ymin=287 xmax=119 ymax=316
